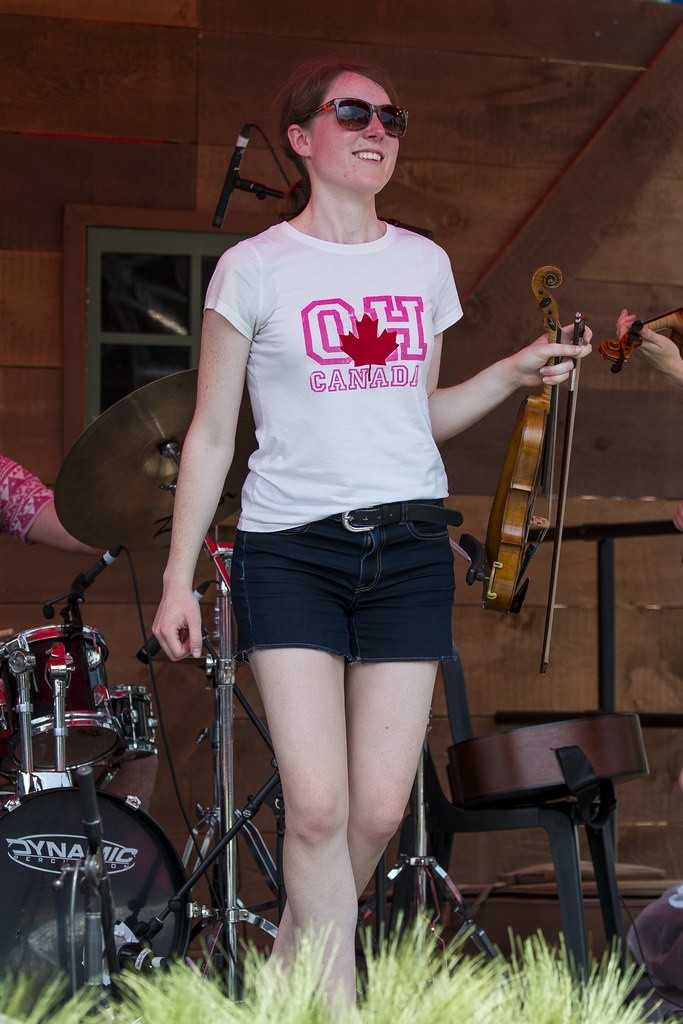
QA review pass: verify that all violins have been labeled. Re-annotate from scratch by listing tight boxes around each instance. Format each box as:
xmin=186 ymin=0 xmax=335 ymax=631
xmin=598 ymin=306 xmax=683 ymax=374
xmin=480 ymin=266 xmax=563 ymax=614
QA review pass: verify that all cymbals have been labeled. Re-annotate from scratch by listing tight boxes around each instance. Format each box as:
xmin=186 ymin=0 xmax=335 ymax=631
xmin=53 ymin=367 xmax=260 ymax=552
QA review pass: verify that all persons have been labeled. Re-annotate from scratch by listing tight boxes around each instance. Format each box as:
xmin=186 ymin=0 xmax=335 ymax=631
xmin=149 ymin=56 xmax=595 ymax=1024
xmin=0 ymin=455 xmax=108 ymax=557
xmin=614 ymin=308 xmax=683 ymax=390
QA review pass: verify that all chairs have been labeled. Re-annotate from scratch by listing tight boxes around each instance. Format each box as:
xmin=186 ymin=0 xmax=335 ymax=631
xmin=389 ymin=643 xmax=628 ymax=985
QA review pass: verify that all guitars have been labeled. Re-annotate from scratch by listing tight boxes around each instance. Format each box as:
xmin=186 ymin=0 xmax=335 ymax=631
xmin=446 ymin=712 xmax=651 ymax=807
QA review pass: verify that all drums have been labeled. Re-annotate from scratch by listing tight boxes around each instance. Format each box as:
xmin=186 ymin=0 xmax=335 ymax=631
xmin=0 ymin=642 xmax=14 ymax=744
xmin=0 ymin=623 xmax=121 ymax=773
xmin=90 ymin=684 xmax=157 ymax=765
xmin=0 ymin=787 xmax=192 ymax=1016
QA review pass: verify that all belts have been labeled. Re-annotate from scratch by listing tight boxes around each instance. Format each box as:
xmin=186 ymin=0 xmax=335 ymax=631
xmin=335 ymin=502 xmax=465 ymax=532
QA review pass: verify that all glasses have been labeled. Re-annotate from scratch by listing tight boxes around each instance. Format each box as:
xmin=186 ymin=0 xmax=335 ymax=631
xmin=306 ymin=95 xmax=409 ymax=140
